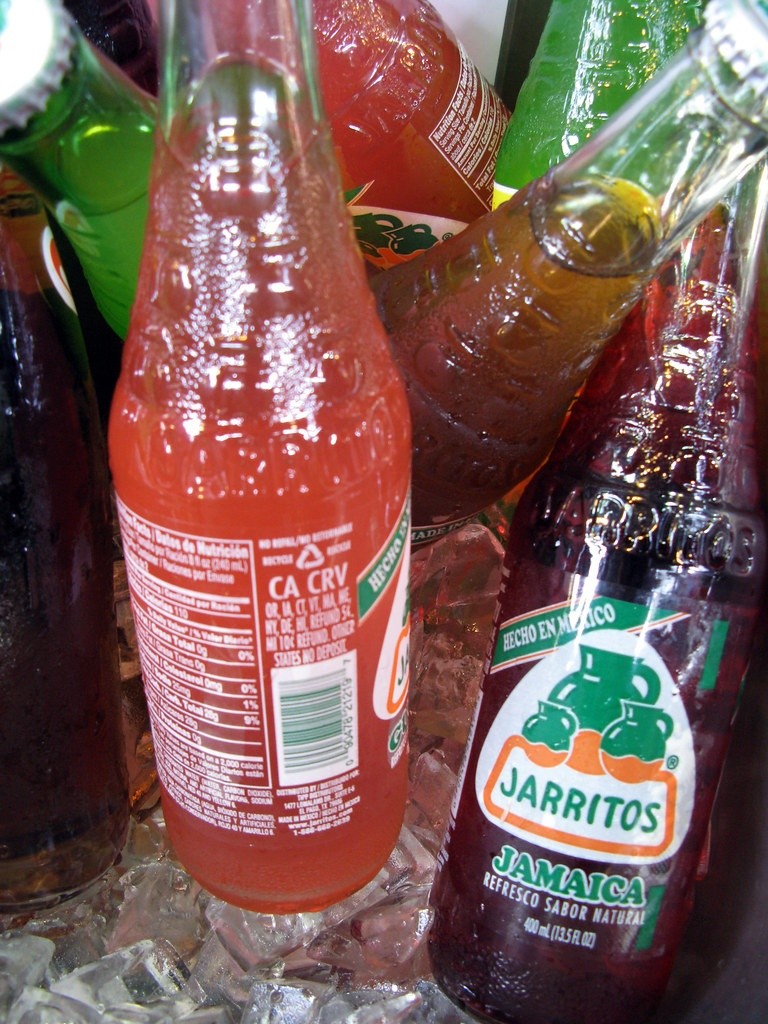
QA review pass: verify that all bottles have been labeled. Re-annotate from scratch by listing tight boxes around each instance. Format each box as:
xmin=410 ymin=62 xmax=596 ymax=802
xmin=0 ymin=0 xmax=768 ymax=1022
